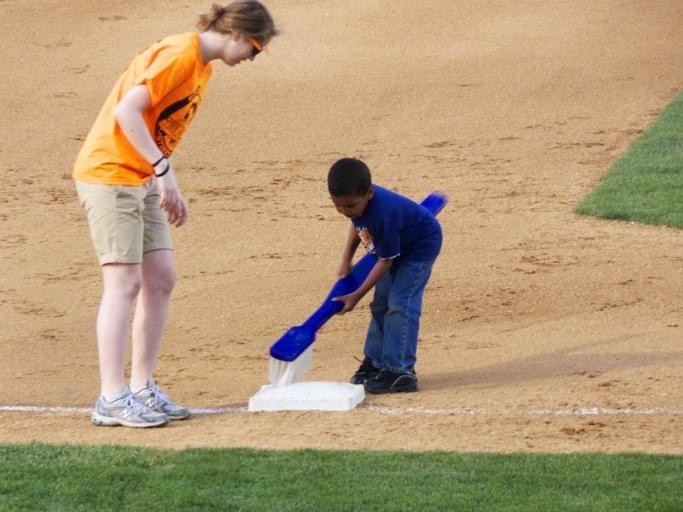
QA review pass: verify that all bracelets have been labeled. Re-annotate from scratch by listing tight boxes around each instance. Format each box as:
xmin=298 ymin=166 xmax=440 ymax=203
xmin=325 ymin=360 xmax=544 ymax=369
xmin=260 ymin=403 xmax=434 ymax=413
xmin=154 ymin=163 xmax=171 ymax=177
xmin=150 ymin=155 xmax=165 ymax=167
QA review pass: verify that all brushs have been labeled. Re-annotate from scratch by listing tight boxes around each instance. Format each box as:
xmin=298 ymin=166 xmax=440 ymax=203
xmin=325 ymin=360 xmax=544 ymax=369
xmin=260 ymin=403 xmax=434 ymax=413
xmin=269 ymin=190 xmax=448 ymax=389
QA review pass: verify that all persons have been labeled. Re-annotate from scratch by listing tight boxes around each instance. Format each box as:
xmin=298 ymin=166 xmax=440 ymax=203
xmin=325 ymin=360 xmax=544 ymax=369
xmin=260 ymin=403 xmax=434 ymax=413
xmin=326 ymin=157 xmax=442 ymax=393
xmin=74 ymin=0 xmax=276 ymax=430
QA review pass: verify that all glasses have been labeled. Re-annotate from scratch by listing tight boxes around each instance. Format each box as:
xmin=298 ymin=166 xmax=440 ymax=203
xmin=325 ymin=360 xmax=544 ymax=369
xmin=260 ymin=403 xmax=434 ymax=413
xmin=248 ymin=38 xmax=263 ymax=55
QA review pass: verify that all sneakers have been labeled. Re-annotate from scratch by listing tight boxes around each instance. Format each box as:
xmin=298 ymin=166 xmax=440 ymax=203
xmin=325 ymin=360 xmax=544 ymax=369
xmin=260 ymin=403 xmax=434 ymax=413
xmin=92 ymin=380 xmax=189 ymax=428
xmin=352 ymin=358 xmax=417 ymax=393
